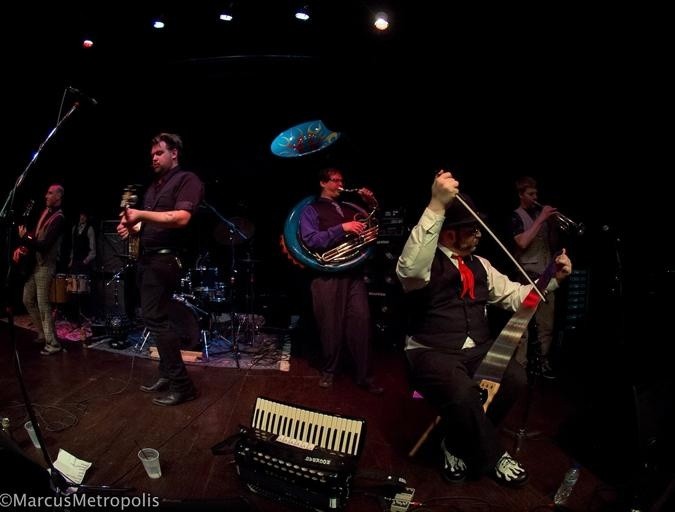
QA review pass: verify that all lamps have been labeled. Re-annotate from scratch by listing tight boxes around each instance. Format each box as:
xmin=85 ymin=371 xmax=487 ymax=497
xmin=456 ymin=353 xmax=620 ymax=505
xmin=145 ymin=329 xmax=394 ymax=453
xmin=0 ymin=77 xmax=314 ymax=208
xmin=294 ymin=8 xmax=311 ymax=20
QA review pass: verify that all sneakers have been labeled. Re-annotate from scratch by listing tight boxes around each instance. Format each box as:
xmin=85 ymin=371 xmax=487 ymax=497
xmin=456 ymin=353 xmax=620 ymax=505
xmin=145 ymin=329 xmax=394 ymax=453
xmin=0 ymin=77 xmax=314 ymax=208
xmin=40 ymin=343 xmax=61 ymax=356
xmin=535 ymin=360 xmax=558 ymax=381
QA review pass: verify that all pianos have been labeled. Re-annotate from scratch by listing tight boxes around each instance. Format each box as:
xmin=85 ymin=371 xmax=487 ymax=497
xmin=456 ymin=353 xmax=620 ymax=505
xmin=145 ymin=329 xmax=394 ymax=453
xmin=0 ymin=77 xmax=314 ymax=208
xmin=234 ymin=395 xmax=369 ymax=476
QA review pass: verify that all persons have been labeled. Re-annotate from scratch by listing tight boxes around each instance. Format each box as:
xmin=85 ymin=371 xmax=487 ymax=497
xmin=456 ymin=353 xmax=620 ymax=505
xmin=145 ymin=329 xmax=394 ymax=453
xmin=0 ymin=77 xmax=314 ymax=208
xmin=299 ymin=165 xmax=385 ymax=396
xmin=12 ymin=185 xmax=66 ymax=354
xmin=68 ymin=211 xmax=96 ymax=325
xmin=510 ymin=176 xmax=557 ymax=379
xmin=395 ymin=169 xmax=572 ymax=488
xmin=116 ymin=131 xmax=205 ymax=406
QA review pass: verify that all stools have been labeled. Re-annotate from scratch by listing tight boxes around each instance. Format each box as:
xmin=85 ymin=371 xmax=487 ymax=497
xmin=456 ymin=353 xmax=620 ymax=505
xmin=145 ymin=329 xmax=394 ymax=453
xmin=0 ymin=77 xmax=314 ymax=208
xmin=409 ymin=391 xmax=440 ymax=457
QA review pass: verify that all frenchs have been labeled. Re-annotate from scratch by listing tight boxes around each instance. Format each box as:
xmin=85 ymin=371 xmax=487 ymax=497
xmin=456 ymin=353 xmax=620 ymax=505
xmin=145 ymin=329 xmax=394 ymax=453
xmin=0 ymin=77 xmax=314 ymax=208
xmin=270 ymin=119 xmax=383 ymax=273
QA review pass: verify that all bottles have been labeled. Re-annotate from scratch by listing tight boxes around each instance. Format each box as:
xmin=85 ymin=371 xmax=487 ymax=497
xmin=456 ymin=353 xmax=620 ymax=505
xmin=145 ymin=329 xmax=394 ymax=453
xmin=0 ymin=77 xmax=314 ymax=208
xmin=553 ymin=462 xmax=582 ymax=506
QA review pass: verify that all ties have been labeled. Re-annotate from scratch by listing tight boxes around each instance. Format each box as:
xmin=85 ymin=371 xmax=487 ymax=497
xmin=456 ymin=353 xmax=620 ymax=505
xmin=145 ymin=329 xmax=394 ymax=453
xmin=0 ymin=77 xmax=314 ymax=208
xmin=332 ymin=201 xmax=346 ymax=219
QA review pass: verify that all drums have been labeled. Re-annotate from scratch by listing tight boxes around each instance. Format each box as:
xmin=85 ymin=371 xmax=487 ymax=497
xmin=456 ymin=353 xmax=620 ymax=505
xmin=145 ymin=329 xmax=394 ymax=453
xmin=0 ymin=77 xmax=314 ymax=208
xmin=48 ymin=272 xmax=90 ymax=303
xmin=173 ymin=266 xmax=228 ymax=303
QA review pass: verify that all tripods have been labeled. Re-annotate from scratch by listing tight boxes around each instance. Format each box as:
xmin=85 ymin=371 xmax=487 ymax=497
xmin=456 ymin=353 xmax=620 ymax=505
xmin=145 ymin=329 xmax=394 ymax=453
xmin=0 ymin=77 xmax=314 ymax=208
xmin=0 ymin=133 xmax=136 ymax=494
xmin=196 ymin=202 xmax=265 ymax=369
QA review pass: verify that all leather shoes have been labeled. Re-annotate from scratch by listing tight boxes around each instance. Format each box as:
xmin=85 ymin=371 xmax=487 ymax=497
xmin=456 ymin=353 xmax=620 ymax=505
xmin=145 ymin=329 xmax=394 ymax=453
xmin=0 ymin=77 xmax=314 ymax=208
xmin=139 ymin=377 xmax=169 ymax=393
xmin=152 ymin=385 xmax=198 ymax=407
xmin=319 ymin=368 xmax=336 ymax=389
xmin=353 ymin=375 xmax=385 ymax=397
xmin=489 ymin=447 xmax=530 ymax=487
xmin=440 ymin=438 xmax=468 ymax=485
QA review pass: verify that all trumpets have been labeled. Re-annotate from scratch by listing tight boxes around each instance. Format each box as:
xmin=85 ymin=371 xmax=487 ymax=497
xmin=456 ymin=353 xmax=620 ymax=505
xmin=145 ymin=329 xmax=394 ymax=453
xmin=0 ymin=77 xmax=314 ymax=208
xmin=532 ymin=200 xmax=587 ymax=239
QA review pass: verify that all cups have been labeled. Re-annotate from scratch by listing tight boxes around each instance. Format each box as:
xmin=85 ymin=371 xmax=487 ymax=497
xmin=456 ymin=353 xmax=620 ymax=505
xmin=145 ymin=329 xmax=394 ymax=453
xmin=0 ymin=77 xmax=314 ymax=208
xmin=24 ymin=420 xmax=44 ymax=448
xmin=137 ymin=449 xmax=165 ymax=483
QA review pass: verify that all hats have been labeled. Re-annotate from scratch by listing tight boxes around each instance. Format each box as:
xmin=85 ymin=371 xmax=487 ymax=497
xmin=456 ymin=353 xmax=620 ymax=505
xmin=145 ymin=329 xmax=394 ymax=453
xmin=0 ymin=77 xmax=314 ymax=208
xmin=443 ymin=192 xmax=482 ymax=231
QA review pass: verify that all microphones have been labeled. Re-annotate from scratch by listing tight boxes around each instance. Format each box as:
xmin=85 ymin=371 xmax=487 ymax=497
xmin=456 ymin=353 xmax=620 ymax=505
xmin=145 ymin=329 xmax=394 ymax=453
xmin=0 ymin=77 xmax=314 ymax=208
xmin=602 ymin=224 xmax=621 ymax=243
xmin=67 ymin=86 xmax=98 ymax=106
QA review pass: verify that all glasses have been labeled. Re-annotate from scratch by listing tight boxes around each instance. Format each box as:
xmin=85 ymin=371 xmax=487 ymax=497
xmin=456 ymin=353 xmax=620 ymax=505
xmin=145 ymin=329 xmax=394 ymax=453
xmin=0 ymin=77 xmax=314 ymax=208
xmin=327 ymin=177 xmax=343 ymax=183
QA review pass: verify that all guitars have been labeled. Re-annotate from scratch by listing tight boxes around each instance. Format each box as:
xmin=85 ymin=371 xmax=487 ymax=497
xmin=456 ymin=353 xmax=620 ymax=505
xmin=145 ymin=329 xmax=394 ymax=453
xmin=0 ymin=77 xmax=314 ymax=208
xmin=120 ymin=184 xmax=141 ymax=262
